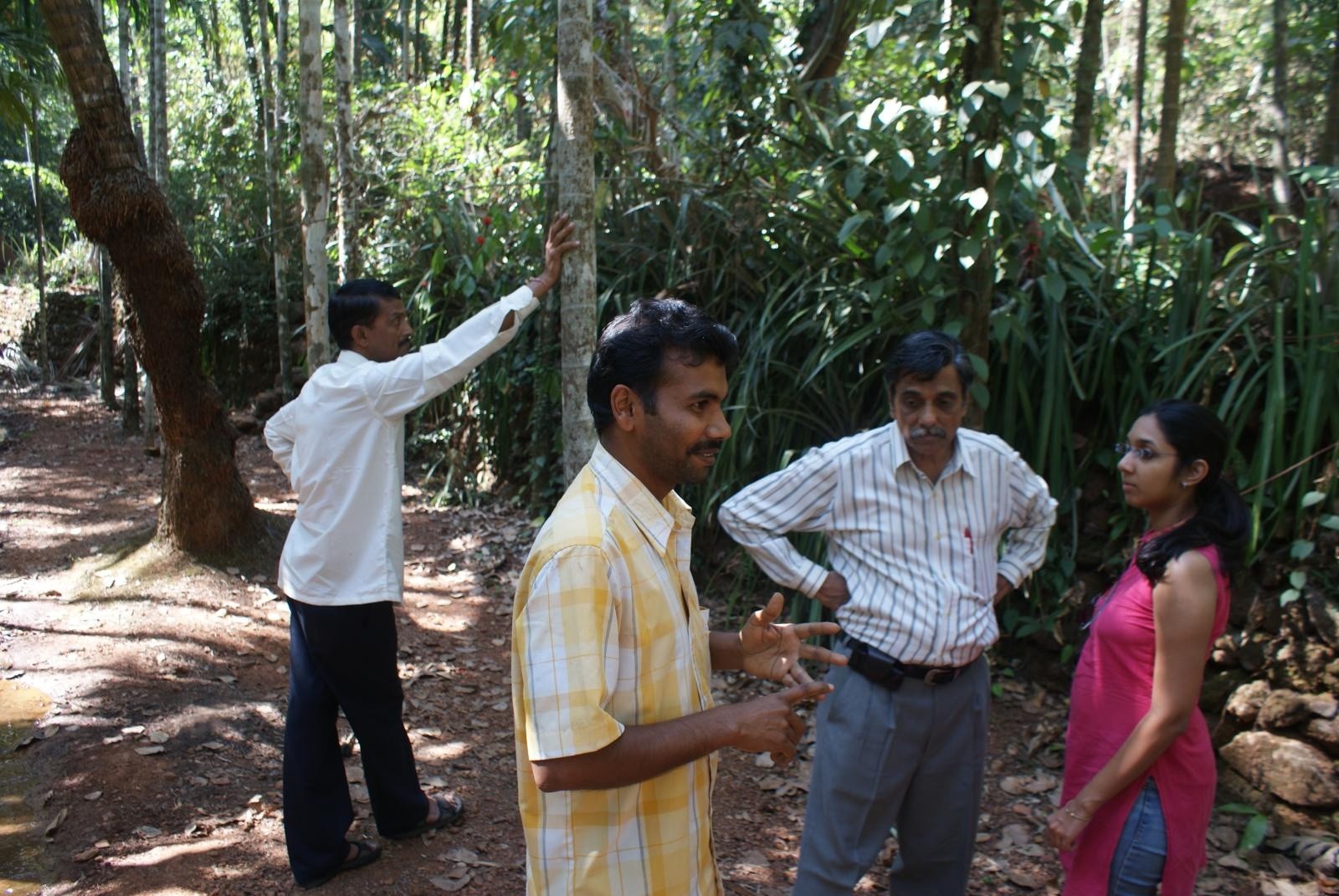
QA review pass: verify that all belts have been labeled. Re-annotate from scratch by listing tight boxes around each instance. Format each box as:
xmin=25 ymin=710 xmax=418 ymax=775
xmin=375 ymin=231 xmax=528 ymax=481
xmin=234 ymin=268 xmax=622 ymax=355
xmin=835 ymin=630 xmax=976 ymax=688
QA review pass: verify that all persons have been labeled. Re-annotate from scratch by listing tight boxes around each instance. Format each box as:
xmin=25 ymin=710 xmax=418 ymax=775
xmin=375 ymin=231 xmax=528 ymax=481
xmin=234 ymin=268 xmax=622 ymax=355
xmin=510 ymin=297 xmax=850 ymax=896
xmin=718 ymin=329 xmax=1059 ymax=896
xmin=1045 ymin=399 xmax=1233 ymax=895
xmin=264 ymin=212 xmax=581 ymax=892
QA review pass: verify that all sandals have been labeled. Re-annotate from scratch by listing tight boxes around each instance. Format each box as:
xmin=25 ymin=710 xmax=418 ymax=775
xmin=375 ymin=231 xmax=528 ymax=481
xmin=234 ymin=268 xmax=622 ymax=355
xmin=295 ymin=840 xmax=382 ymax=889
xmin=390 ymin=793 xmax=463 ymax=839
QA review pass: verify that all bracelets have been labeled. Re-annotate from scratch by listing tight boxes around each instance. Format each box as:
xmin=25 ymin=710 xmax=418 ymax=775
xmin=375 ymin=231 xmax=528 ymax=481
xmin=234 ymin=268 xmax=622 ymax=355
xmin=1064 ymin=797 xmax=1096 ymax=822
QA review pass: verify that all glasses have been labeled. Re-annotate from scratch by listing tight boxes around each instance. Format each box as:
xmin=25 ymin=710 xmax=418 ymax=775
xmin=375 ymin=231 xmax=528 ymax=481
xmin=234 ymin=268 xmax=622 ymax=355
xmin=1115 ymin=443 xmax=1183 ymax=464
